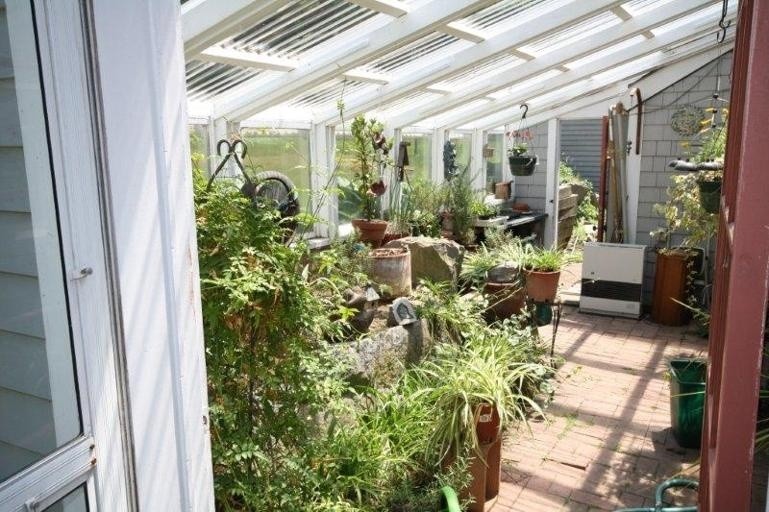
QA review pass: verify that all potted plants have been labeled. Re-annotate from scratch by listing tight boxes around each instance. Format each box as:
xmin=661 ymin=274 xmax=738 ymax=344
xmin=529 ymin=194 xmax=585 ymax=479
xmin=336 ymin=331 xmax=560 ymax=510
xmin=649 ymin=101 xmax=728 ymax=257
xmin=504 ymin=121 xmax=543 ymax=180
xmin=338 ymin=110 xmax=397 ymax=246
xmin=461 ymin=232 xmax=583 ymax=323
